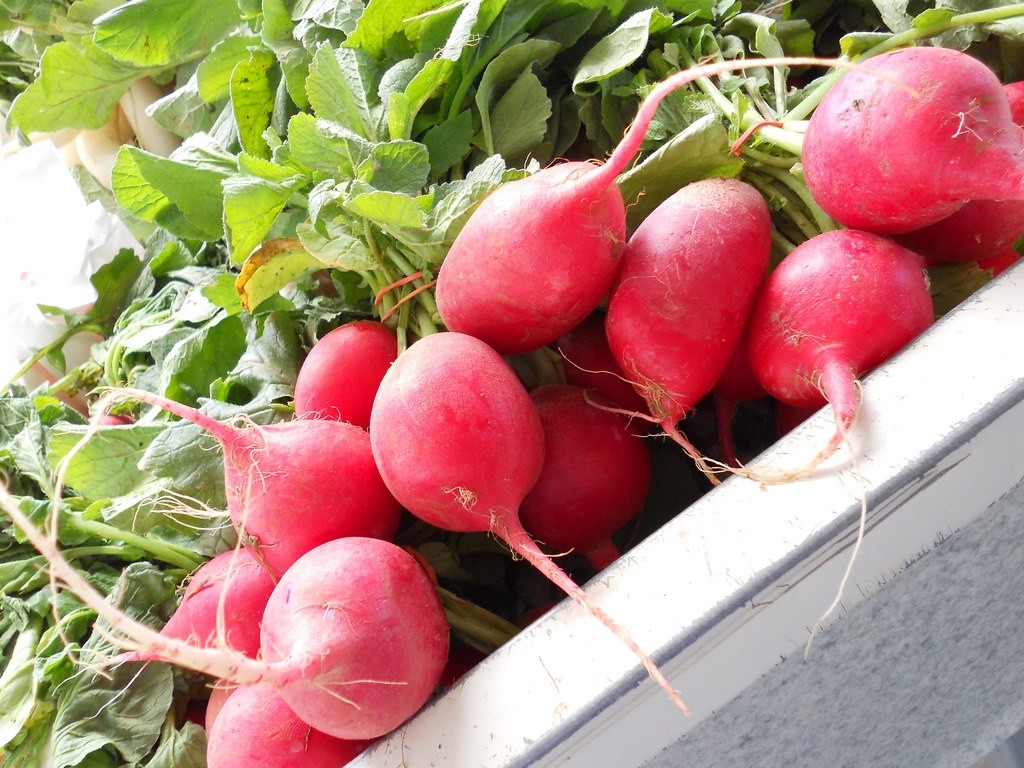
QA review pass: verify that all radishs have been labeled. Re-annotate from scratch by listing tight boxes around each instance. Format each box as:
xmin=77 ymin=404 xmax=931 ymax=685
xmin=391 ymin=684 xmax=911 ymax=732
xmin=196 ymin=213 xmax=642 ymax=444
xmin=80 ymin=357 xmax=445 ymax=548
xmin=0 ymin=74 xmax=179 ymax=418
